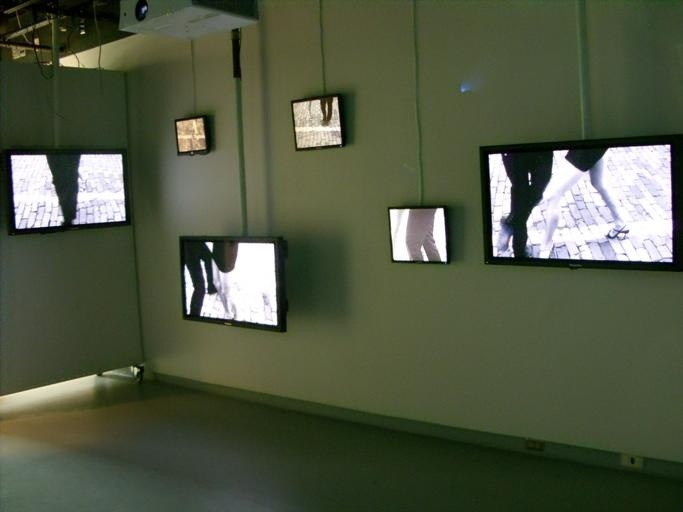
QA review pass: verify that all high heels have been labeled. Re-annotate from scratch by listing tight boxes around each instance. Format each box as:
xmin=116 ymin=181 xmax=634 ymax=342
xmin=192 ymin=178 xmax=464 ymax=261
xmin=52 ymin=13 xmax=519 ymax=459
xmin=604 ymin=223 xmax=629 ymax=239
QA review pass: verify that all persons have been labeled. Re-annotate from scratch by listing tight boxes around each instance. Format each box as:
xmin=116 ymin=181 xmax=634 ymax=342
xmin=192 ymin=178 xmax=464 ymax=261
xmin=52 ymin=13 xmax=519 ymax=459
xmin=536 ymin=147 xmax=629 ymax=259
xmin=404 ymin=209 xmax=441 ymax=262
xmin=497 ymin=152 xmax=554 ymax=259
xmin=182 ymin=241 xmax=217 ymax=320
xmin=211 ymin=240 xmax=237 ymax=321
xmin=318 ymin=97 xmax=334 ymax=127
xmin=45 ymin=154 xmax=81 ymax=229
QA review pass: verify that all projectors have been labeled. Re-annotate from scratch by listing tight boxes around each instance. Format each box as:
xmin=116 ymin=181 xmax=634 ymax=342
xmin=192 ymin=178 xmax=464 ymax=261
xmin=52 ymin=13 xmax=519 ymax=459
xmin=118 ymin=0 xmax=259 ymax=38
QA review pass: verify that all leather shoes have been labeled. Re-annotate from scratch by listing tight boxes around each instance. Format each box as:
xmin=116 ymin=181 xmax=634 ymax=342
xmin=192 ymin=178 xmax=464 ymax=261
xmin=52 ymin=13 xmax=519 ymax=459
xmin=497 ymin=215 xmax=512 ymax=252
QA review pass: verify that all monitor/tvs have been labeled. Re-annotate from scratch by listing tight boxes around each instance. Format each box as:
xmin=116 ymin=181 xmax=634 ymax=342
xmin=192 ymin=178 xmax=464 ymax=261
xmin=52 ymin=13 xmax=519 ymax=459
xmin=387 ymin=206 xmax=452 ymax=265
xmin=174 ymin=115 xmax=210 ymax=156
xmin=178 ymin=235 xmax=286 ymax=333
xmin=0 ymin=146 xmax=131 ymax=236
xmin=480 ymin=131 xmax=683 ymax=274
xmin=290 ymin=94 xmax=346 ymax=152
